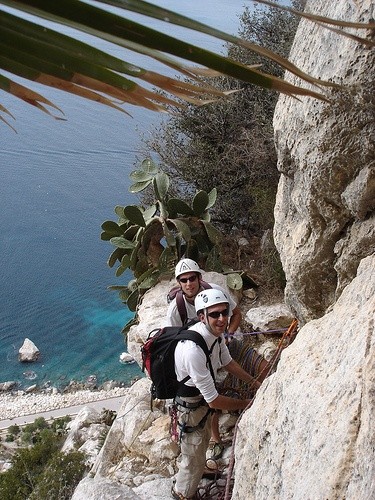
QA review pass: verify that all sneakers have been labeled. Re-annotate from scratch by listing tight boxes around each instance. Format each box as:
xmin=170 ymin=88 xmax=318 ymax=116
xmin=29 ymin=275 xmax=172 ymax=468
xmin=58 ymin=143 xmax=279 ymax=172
xmin=171 ymin=486 xmax=187 ymax=500
xmin=212 ymin=442 xmax=224 ymax=457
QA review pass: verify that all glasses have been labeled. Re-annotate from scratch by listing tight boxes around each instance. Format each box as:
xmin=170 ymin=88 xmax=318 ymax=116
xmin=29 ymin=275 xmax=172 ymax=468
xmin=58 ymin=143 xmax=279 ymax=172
xmin=207 ymin=309 xmax=229 ymax=318
xmin=180 ymin=275 xmax=197 ymax=283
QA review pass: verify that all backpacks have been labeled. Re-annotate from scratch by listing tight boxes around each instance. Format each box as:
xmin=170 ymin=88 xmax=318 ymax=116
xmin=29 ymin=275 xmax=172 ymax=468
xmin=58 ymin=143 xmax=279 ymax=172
xmin=141 ymin=320 xmax=217 ymax=411
xmin=168 ymin=281 xmax=215 ymax=330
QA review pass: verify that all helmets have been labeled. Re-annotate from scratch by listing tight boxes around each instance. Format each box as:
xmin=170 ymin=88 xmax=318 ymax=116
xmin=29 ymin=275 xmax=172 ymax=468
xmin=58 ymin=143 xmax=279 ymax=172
xmin=195 ymin=289 xmax=230 ymax=313
xmin=175 ymin=258 xmax=201 ymax=280
